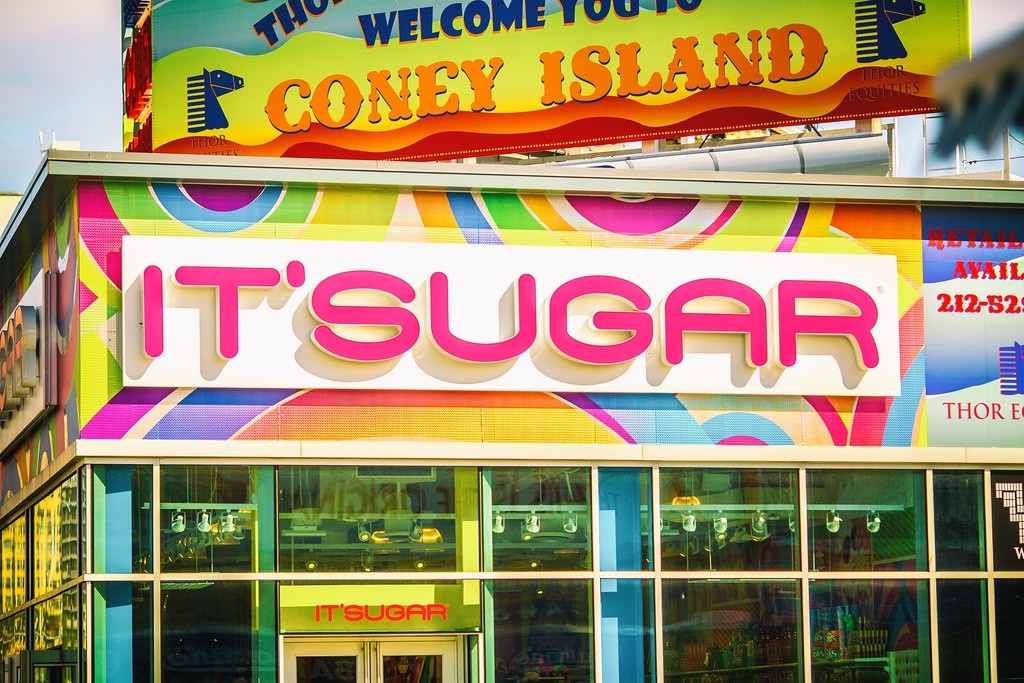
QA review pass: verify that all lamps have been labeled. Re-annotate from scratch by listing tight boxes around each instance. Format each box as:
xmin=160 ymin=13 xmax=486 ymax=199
xmin=494 ymin=512 xmax=578 ymax=568
xmin=282 ymin=521 xmax=327 ymax=536
xmin=360 ymin=517 xmax=401 ymax=570
xmin=826 ymin=510 xmax=840 ymax=532
xmin=304 ymin=551 xmax=318 ymax=571
xmin=641 ymin=520 xmax=679 ymax=537
xmin=867 ymin=510 xmax=880 ymax=533
xmin=410 ymin=512 xmax=445 ymax=570
xmin=134 ymin=509 xmax=245 ymax=573
xmin=680 ymin=508 xmax=771 ymax=556
xmin=671 ymin=469 xmax=702 ymax=506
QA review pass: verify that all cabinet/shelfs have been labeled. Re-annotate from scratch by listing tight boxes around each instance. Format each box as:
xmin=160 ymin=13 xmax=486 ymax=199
xmin=665 ymin=646 xmax=921 ymax=682
